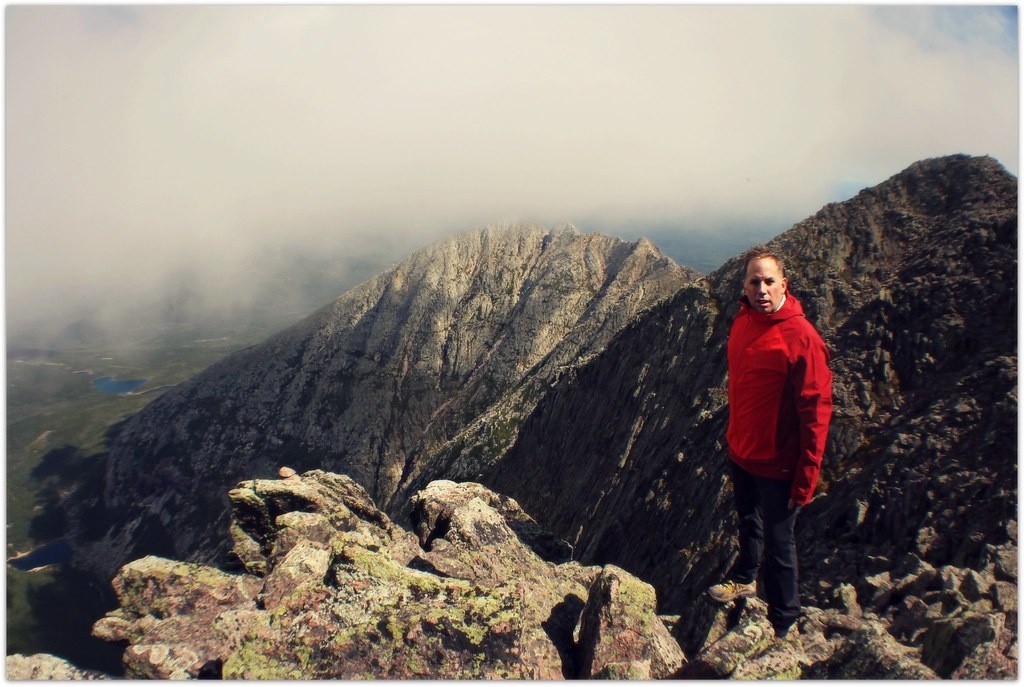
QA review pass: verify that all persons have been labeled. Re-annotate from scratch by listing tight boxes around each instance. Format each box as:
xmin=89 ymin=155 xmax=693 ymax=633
xmin=707 ymin=247 xmax=834 ymax=626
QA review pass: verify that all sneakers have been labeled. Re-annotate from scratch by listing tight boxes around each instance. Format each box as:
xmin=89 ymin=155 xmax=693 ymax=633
xmin=708 ymin=578 xmax=757 ymax=602
